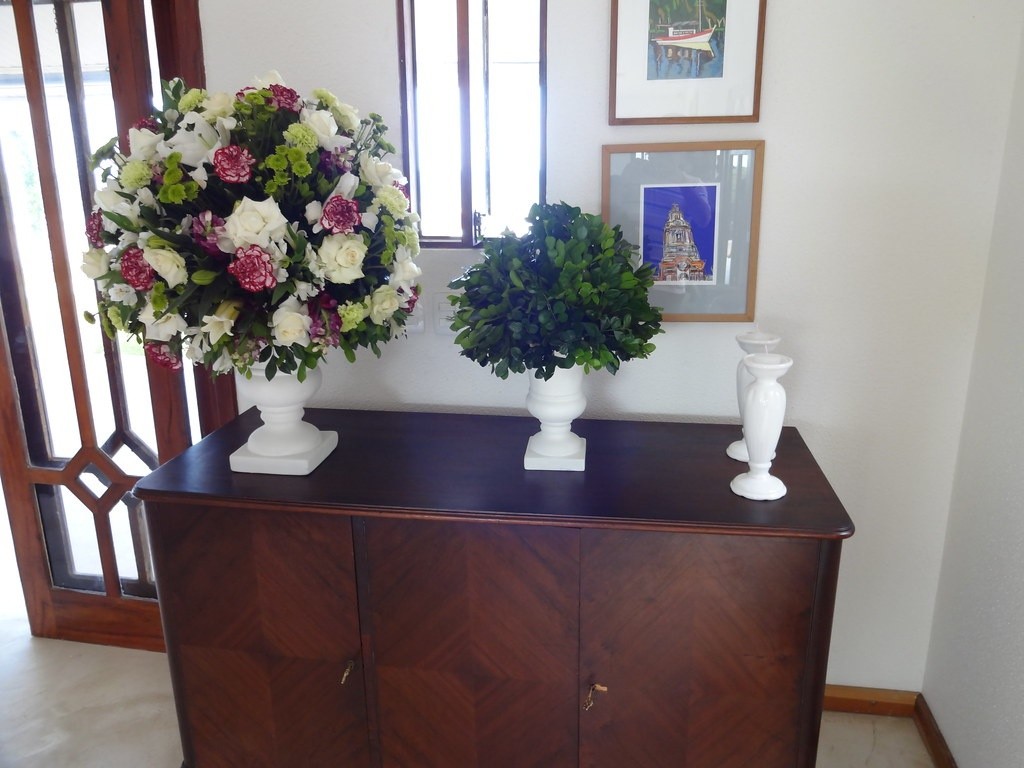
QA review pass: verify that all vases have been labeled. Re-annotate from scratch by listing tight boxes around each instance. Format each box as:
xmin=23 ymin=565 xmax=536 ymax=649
xmin=725 ymin=331 xmax=784 ymax=462
xmin=229 ymin=358 xmax=339 ymax=475
xmin=730 ymin=352 xmax=793 ymax=501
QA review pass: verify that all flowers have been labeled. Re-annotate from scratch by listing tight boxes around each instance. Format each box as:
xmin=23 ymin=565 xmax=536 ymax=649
xmin=86 ymin=77 xmax=421 ymax=382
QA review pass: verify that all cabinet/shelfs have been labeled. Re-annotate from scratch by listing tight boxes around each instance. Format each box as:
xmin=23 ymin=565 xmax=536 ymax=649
xmin=130 ymin=405 xmax=853 ymax=768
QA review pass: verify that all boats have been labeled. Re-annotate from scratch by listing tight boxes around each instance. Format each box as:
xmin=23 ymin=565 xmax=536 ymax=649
xmin=649 ymin=0 xmax=719 ymax=42
xmin=653 ymin=42 xmax=714 ymax=76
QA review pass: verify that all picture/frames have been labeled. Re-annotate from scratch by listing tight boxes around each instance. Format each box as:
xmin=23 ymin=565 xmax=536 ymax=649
xmin=608 ymin=0 xmax=766 ymax=126
xmin=600 ymin=139 xmax=765 ymax=323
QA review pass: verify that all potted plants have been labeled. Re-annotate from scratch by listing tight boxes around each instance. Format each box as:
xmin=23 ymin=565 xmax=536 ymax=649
xmin=448 ymin=199 xmax=666 ymax=473
